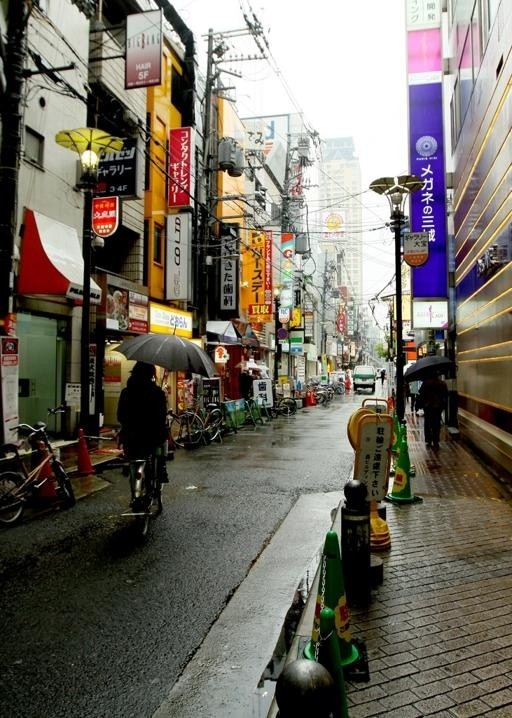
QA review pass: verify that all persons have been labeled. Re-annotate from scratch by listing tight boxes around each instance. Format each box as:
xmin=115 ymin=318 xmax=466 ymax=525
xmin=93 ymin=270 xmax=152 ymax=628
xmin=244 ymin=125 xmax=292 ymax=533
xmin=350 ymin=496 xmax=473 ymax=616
xmin=408 ymin=380 xmax=423 ymax=413
xmin=417 ymin=368 xmax=449 ymax=447
xmin=115 ymin=360 xmax=169 ymax=518
xmin=380 ymin=370 xmax=385 ymax=385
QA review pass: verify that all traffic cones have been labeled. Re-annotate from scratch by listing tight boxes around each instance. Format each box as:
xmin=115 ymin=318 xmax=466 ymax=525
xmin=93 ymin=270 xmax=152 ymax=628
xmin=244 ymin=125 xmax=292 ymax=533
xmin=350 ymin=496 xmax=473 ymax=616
xmin=36 ymin=438 xmax=62 ymax=505
xmin=385 ymin=387 xmax=424 ymax=505
xmin=311 ymin=605 xmax=354 ymax=718
xmin=294 ymin=528 xmax=373 ymax=683
xmin=72 ymin=423 xmax=98 ymax=476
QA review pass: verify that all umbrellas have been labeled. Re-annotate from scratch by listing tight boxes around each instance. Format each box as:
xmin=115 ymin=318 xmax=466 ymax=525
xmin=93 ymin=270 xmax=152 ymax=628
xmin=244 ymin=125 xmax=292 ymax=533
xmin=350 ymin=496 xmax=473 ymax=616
xmin=404 ymin=355 xmax=456 ymax=381
xmin=109 ymin=326 xmax=216 ymax=391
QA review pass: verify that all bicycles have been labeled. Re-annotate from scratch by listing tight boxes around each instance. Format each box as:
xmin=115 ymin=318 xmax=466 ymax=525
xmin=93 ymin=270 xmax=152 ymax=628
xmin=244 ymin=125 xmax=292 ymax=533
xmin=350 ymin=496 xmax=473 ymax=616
xmin=2 ymin=402 xmax=78 ymax=521
xmin=119 ymin=408 xmax=176 ymax=536
xmin=164 ymin=373 xmax=345 ymax=449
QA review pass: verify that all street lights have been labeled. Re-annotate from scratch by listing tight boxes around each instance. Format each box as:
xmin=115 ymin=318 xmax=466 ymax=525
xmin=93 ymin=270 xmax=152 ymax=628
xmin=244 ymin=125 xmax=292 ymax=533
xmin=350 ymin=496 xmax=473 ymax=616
xmin=273 ymin=282 xmax=289 ymax=405
xmin=369 ymin=175 xmax=423 ymax=422
xmin=54 ymin=125 xmax=128 ymax=444
xmin=339 ymin=334 xmax=344 ymax=370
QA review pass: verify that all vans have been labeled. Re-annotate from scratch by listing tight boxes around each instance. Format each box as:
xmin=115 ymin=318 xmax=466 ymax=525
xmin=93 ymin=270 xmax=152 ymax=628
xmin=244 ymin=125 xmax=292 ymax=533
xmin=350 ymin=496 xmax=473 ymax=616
xmin=352 ymin=364 xmax=376 ymax=392
xmin=375 ymin=367 xmax=383 ymax=378
